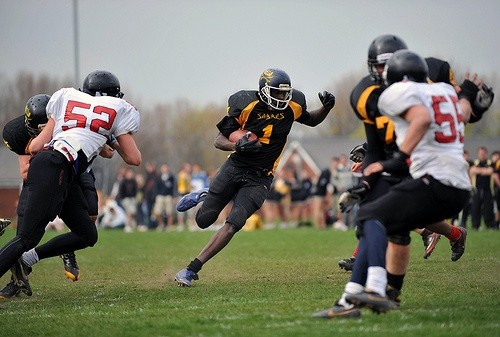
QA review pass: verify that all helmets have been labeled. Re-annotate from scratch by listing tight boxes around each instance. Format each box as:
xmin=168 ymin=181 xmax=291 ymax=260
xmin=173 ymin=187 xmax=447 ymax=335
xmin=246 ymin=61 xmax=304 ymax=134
xmin=259 ymin=68 xmax=293 ymax=111
xmin=83 ymin=71 xmax=124 ymax=98
xmin=367 ymin=35 xmax=409 ymax=84
xmin=24 ymin=94 xmax=51 ymax=134
xmin=383 ymin=49 xmax=429 ymax=88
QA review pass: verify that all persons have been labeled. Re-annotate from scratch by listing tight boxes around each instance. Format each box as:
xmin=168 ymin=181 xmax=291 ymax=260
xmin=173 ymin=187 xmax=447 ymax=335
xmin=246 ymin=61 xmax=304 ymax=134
xmin=313 ymin=34 xmax=495 ymax=319
xmin=174 ymin=69 xmax=335 ymax=287
xmin=43 ymin=147 xmax=500 ymax=235
xmin=0 ymin=70 xmax=142 ymax=300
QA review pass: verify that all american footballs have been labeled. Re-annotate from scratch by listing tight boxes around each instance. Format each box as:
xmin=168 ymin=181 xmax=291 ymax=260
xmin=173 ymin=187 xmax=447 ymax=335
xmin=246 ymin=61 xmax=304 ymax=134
xmin=229 ymin=129 xmax=261 ymax=146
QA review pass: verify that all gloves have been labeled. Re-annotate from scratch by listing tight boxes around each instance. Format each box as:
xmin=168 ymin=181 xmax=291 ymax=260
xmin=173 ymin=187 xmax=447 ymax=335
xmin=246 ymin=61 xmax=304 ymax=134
xmin=107 ymin=133 xmax=117 ymax=149
xmin=338 ymin=183 xmax=368 ymax=214
xmin=318 ymin=91 xmax=335 ymax=111
xmin=234 ymin=131 xmax=262 ymax=157
xmin=350 ymin=143 xmax=367 ymax=162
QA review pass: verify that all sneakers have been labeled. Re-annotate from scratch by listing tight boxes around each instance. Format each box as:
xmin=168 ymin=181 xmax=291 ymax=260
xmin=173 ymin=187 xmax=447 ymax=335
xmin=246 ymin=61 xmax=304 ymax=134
xmin=174 ymin=267 xmax=196 ymax=288
xmin=10 ymin=255 xmax=32 ymax=297
xmin=61 ymin=252 xmax=80 ymax=282
xmin=423 ymin=233 xmax=441 ymax=259
xmin=0 ymin=282 xmax=20 ymax=300
xmin=387 ymin=297 xmax=401 ymax=308
xmin=338 ymin=257 xmax=356 ymax=270
xmin=313 ymin=305 xmax=360 ymax=318
xmin=450 ymin=227 xmax=468 ymax=261
xmin=346 ymin=291 xmax=389 ymax=314
xmin=177 ymin=188 xmax=209 ymax=212
